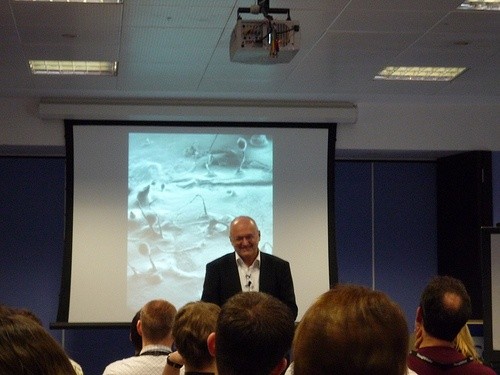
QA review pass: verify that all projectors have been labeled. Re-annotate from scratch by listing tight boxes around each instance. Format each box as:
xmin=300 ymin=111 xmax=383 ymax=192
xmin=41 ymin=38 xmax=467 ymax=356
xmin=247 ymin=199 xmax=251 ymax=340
xmin=230 ymin=20 xmax=299 ymax=64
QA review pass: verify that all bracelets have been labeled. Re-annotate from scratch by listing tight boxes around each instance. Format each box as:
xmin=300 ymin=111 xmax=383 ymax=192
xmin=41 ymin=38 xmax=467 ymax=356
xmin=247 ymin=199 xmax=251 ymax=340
xmin=167 ymin=353 xmax=183 ymax=369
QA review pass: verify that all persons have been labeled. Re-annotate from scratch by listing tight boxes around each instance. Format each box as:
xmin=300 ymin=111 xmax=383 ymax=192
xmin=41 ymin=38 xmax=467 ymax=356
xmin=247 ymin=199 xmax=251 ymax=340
xmin=200 ymin=216 xmax=298 ymax=326
xmin=0 ymin=275 xmax=499 ymax=375
